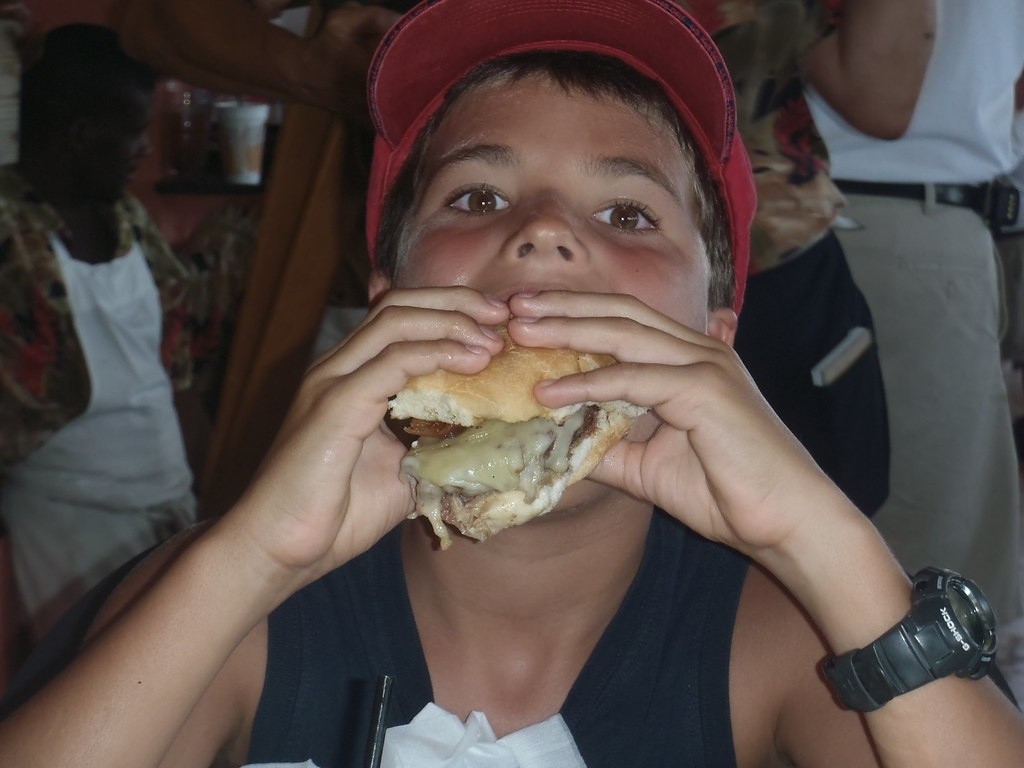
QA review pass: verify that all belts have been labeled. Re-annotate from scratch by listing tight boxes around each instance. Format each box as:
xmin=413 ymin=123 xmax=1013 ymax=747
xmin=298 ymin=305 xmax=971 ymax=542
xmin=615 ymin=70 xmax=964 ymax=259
xmin=834 ymin=179 xmax=988 ymax=215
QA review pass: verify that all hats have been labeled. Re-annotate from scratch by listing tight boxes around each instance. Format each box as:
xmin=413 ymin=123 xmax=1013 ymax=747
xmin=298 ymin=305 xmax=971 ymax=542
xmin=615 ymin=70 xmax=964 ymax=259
xmin=367 ymin=0 xmax=758 ymax=316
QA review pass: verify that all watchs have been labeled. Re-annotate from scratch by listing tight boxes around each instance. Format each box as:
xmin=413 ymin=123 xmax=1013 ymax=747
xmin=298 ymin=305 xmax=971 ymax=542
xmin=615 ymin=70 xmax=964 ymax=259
xmin=817 ymin=564 xmax=999 ymax=713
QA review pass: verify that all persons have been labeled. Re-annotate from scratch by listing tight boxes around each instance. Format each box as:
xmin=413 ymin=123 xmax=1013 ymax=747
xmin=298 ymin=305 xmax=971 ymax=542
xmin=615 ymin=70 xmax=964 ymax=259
xmin=791 ymin=0 xmax=1022 ymax=718
xmin=2 ymin=0 xmax=1023 ymax=767
xmin=661 ymin=0 xmax=941 ymax=521
xmin=1 ymin=23 xmax=201 ymax=721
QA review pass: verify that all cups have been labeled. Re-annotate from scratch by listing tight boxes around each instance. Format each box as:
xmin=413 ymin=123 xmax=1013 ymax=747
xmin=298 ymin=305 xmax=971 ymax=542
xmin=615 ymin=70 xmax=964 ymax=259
xmin=158 ymin=89 xmax=211 ymax=184
xmin=216 ymin=100 xmax=269 ymax=188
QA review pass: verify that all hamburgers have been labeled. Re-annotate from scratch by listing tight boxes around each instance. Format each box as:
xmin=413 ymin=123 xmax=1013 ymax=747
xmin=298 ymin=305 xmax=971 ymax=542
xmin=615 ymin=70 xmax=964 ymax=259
xmin=384 ymin=315 xmax=647 ymax=540
